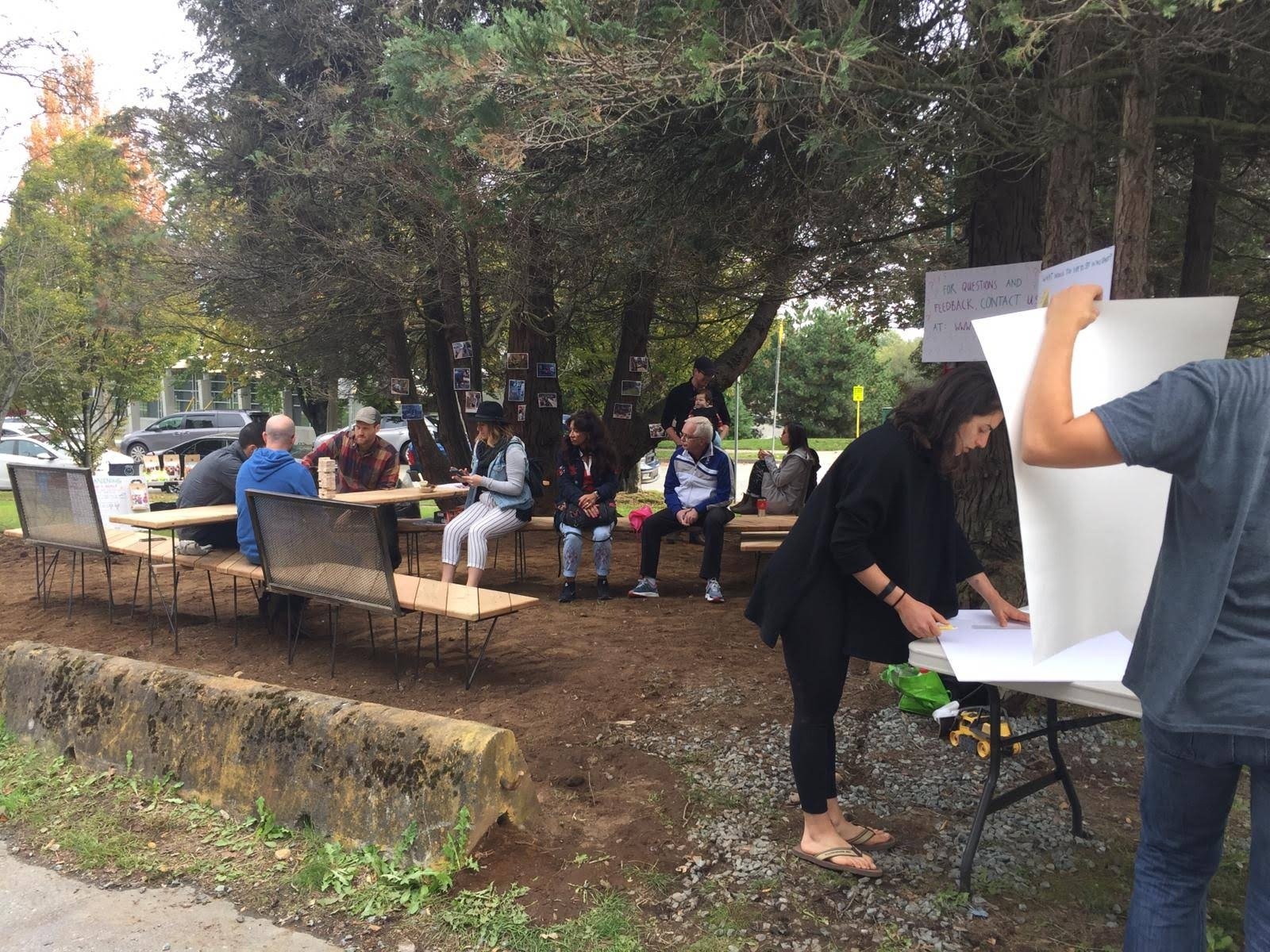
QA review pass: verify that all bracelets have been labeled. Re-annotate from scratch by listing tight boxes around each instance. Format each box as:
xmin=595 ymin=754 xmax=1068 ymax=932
xmin=877 ymin=580 xmax=897 ymax=600
xmin=891 ymin=591 xmax=906 ymax=608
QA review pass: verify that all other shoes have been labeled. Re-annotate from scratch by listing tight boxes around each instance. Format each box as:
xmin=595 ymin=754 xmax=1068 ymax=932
xmin=665 ymin=530 xmax=680 ymax=543
xmin=689 ymin=532 xmax=705 ymax=545
xmin=257 ymin=590 xmax=312 ymax=638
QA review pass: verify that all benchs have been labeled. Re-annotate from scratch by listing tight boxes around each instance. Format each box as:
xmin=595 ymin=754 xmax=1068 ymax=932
xmin=687 ymin=515 xmax=800 ymax=585
xmin=513 ymin=517 xmax=640 ymax=586
xmin=4 ymin=524 xmax=539 ymax=691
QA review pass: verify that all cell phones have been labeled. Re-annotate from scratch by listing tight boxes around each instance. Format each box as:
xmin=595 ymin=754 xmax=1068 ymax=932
xmin=450 ymin=469 xmax=464 ymax=476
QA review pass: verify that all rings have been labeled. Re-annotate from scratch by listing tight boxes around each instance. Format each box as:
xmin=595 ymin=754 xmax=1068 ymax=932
xmin=587 ymin=502 xmax=589 ymax=505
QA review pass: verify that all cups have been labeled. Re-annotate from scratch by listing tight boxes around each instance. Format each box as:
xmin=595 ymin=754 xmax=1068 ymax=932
xmin=757 ymin=497 xmax=766 ymax=517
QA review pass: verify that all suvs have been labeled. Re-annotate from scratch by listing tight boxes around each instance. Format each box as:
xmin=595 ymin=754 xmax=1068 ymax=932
xmin=121 ymin=409 xmax=271 ymax=463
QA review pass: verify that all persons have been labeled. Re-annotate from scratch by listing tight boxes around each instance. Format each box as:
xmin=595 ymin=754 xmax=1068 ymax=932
xmin=236 ymin=414 xmax=362 ymax=638
xmin=661 ymin=356 xmax=731 ymax=451
xmin=301 ymin=406 xmax=403 ymax=573
xmin=1019 ymin=285 xmax=1270 ymax=952
xmin=629 ymin=416 xmax=735 ymax=602
xmin=743 ymin=366 xmax=1032 ymax=877
xmin=688 ymin=388 xmax=723 ymax=431
xmin=441 ymin=401 xmax=535 ymax=588
xmin=176 ymin=421 xmax=267 ymax=546
xmin=731 ymin=422 xmax=814 ymax=514
xmin=555 ymin=411 xmax=619 ymax=602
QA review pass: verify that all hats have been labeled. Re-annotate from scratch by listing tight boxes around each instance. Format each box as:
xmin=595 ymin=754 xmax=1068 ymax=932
xmin=695 ymin=357 xmax=717 ymax=376
xmin=351 ymin=406 xmax=381 ymax=425
xmin=468 ymin=401 xmax=512 ymax=425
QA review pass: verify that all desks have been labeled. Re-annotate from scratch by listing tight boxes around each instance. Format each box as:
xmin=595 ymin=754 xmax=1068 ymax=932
xmin=110 ymin=483 xmax=470 ymax=654
xmin=909 ymin=636 xmax=1143 ymax=892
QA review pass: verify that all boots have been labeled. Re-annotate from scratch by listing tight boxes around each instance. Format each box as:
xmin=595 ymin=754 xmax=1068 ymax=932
xmin=730 ymin=492 xmax=761 ymax=514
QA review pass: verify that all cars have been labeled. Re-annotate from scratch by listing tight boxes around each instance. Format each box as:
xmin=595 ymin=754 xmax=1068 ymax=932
xmin=311 ymin=413 xmax=438 ymax=466
xmin=562 ymin=414 xmax=660 ymax=484
xmin=1 ymin=416 xmax=148 ymax=495
xmin=144 ymin=432 xmax=239 ymax=493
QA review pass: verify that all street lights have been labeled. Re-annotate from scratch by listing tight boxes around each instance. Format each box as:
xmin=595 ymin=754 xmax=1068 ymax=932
xmin=772 ymin=304 xmax=786 ymax=449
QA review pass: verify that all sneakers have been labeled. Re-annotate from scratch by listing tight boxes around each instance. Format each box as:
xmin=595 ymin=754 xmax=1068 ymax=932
xmin=596 ymin=577 xmax=611 ymax=600
xmin=705 ymin=577 xmax=725 ymax=602
xmin=628 ymin=577 xmax=660 ymax=597
xmin=558 ymin=580 xmax=576 ymax=602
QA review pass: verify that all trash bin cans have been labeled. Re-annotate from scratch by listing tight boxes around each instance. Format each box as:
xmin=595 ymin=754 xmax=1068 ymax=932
xmin=108 ymin=461 xmax=140 ymax=476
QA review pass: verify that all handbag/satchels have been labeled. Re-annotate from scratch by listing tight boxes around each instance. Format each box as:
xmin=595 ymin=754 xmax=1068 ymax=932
xmin=559 ymin=489 xmax=616 ymax=528
xmin=880 ymin=663 xmax=952 ymax=716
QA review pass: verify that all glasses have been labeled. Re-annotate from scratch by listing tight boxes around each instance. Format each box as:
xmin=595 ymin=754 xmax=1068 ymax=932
xmin=699 ymin=370 xmax=715 ymax=379
xmin=679 ymin=430 xmax=701 ymax=441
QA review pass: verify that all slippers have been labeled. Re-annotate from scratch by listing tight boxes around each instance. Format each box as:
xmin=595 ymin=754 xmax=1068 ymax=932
xmin=845 ymin=819 xmax=896 ymax=850
xmin=791 ymin=838 xmax=883 ymax=878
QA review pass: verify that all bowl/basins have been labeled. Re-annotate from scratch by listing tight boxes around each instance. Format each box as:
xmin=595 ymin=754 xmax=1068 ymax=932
xmin=419 ymin=484 xmax=436 ymax=492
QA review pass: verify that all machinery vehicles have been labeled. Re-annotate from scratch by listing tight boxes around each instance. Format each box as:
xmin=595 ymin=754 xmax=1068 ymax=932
xmin=950 ymin=706 xmax=1022 ymax=760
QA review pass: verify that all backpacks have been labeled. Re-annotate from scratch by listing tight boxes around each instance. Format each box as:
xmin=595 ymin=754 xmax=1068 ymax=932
xmin=503 ymin=440 xmax=546 ymax=498
xmin=629 ymin=505 xmax=653 ymax=573
